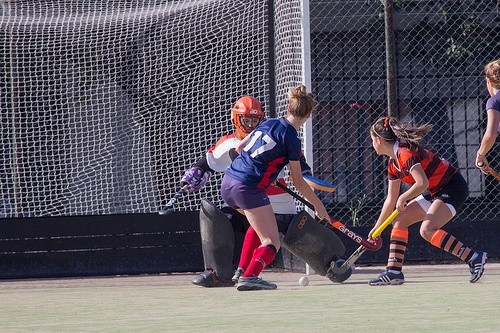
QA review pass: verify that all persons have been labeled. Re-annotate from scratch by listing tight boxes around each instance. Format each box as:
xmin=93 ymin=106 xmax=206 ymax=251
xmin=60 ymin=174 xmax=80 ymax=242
xmin=221 ymin=85 xmax=332 ymax=290
xmin=368 ymin=118 xmax=487 ymax=285
xmin=181 ymin=97 xmax=347 ymax=286
xmin=475 ymin=60 xmax=500 ymax=175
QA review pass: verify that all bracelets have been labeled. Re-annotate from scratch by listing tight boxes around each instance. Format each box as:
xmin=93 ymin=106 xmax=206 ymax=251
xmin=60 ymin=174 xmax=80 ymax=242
xmin=477 ymin=151 xmax=486 ymax=157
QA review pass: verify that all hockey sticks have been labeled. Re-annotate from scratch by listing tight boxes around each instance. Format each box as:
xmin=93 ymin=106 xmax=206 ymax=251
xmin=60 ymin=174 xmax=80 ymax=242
xmin=273 ymin=181 xmax=383 ymax=251
xmin=477 ymin=160 xmax=500 ymax=181
xmin=330 ymin=202 xmax=404 ymax=274
xmin=159 ymin=183 xmax=189 ymax=215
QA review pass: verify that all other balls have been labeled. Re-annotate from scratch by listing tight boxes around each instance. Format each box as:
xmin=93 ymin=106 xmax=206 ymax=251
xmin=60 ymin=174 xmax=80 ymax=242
xmin=299 ymin=277 xmax=309 ymax=286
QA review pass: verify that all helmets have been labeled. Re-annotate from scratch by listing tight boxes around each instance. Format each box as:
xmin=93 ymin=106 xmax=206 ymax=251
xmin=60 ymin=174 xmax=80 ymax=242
xmin=231 ymin=96 xmax=264 ymax=139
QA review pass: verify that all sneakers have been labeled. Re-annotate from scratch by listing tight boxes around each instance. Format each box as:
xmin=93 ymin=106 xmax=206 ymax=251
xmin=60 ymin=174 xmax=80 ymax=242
xmin=368 ymin=270 xmax=404 ymax=286
xmin=237 ymin=276 xmax=277 ymax=291
xmin=232 ymin=269 xmax=245 ymax=281
xmin=469 ymin=251 xmax=488 ymax=283
